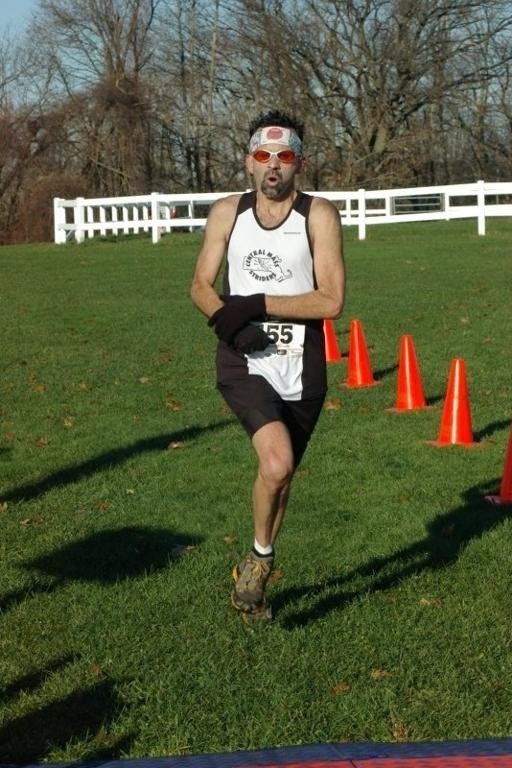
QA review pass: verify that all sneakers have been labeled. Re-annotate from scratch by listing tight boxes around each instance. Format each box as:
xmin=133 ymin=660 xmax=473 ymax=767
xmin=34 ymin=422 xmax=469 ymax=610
xmin=231 ymin=549 xmax=275 ymax=624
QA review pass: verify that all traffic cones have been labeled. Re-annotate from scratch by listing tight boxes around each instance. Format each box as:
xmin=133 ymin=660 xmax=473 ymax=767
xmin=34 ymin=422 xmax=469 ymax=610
xmin=484 ymin=424 xmax=512 ymax=505
xmin=424 ymin=358 xmax=480 ymax=448
xmin=384 ymin=334 xmax=432 ymax=414
xmin=341 ymin=319 xmax=381 ymax=389
xmin=323 ymin=319 xmax=348 ymax=362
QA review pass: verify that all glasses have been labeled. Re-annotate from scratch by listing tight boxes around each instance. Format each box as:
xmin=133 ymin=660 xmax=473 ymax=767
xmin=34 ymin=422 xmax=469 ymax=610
xmin=253 ymin=149 xmax=297 ymax=164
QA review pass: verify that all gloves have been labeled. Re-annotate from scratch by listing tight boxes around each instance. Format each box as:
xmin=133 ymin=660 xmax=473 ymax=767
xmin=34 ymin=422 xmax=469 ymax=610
xmin=207 ymin=293 xmax=270 ymax=354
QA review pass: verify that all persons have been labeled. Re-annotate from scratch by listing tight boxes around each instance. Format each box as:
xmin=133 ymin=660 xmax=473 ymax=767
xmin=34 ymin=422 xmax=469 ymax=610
xmin=190 ymin=110 xmax=346 ymax=625
